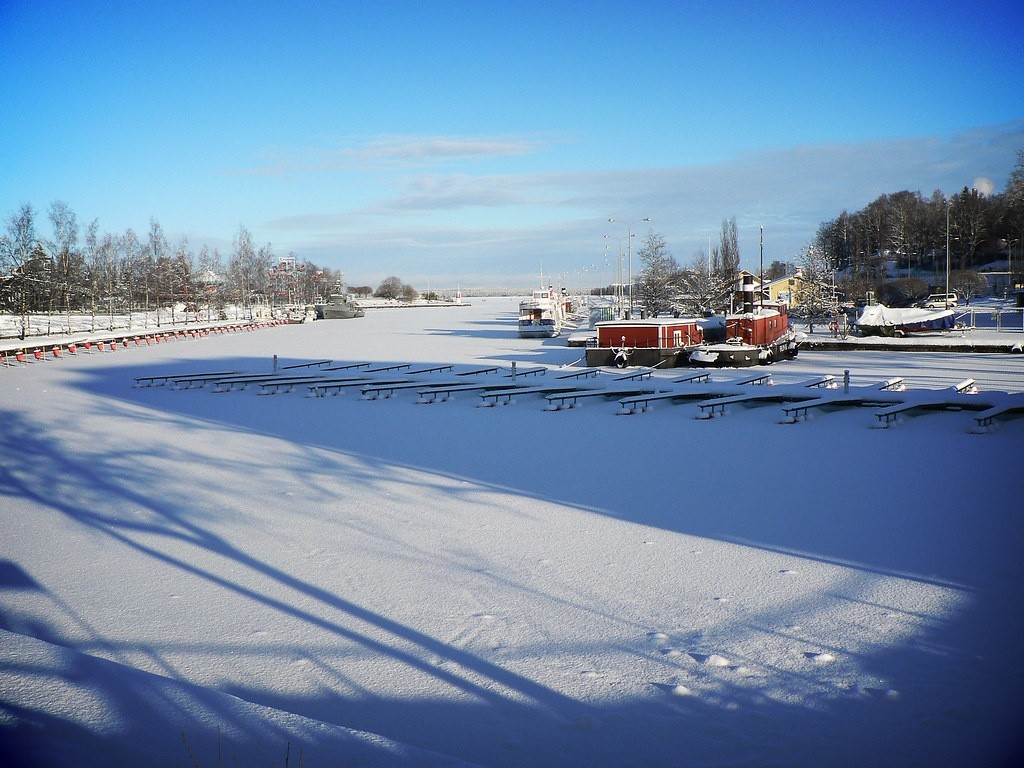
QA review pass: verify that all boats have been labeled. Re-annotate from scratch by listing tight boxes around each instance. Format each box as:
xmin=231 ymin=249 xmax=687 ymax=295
xmin=315 ymin=293 xmax=366 ymax=319
xmin=690 ymin=276 xmax=799 ymax=369
xmin=518 ymin=289 xmax=563 ymax=338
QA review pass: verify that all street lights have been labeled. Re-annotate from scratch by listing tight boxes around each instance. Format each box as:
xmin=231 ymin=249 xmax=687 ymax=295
xmin=608 ymin=217 xmax=651 ymax=314
xmin=1002 ymin=238 xmax=1020 ymax=287
xmin=603 ymin=246 xmax=634 ymax=309
xmin=604 ymin=234 xmax=636 ymax=316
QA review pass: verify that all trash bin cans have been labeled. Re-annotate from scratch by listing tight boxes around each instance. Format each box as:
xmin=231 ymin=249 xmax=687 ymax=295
xmin=1013 ymin=290 xmax=1024 ymax=307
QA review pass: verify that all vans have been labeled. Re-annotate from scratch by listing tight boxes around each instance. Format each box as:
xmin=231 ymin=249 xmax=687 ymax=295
xmin=920 ymin=292 xmax=959 ymax=308
